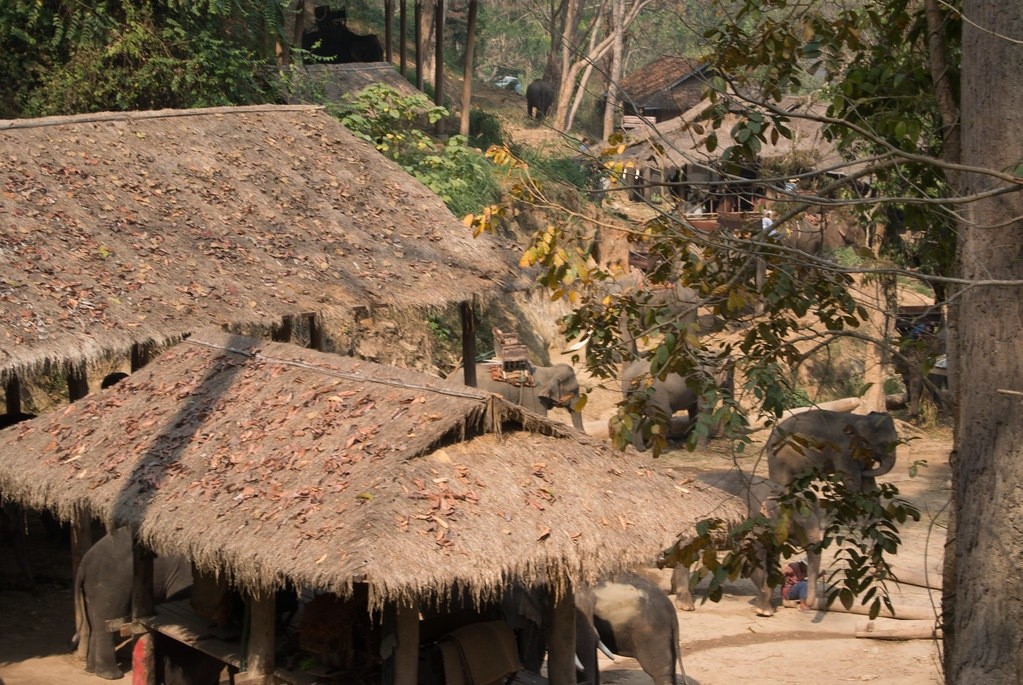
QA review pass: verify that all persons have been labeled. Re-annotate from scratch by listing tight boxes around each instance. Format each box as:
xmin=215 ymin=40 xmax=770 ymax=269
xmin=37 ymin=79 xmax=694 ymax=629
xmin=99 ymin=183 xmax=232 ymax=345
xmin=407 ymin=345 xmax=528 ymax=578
xmin=778 ymin=556 xmax=849 ymax=612
xmin=762 ymin=210 xmax=777 ymax=237
xmin=579 ymin=139 xmax=589 ymax=151
xmin=911 ymin=323 xmax=934 ymax=340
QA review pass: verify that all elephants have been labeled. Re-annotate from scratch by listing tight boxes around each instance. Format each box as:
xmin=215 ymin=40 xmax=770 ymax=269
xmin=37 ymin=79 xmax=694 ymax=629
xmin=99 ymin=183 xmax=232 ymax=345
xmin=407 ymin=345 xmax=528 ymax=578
xmin=71 ymin=526 xmax=686 ymax=685
xmin=452 ymin=281 xmax=735 ymax=452
xmin=659 ymin=410 xmax=897 ymax=617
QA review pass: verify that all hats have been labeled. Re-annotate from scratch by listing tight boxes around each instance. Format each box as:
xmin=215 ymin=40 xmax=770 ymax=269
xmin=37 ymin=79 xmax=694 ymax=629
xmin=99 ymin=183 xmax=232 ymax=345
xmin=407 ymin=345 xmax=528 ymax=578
xmin=801 ymin=555 xmax=808 ymax=566
